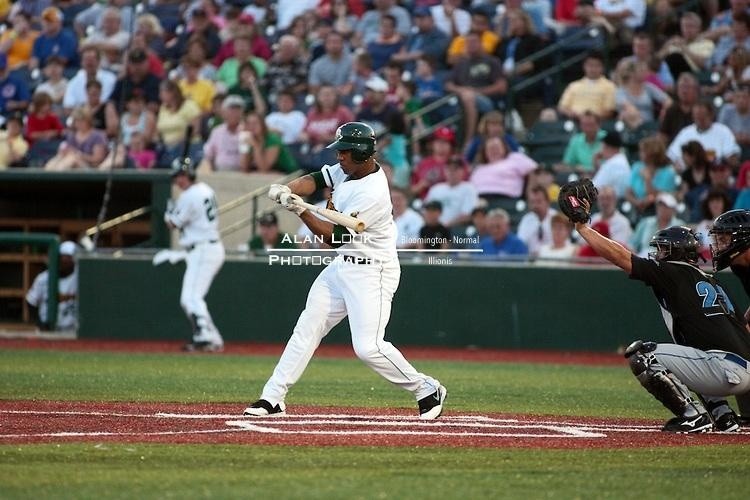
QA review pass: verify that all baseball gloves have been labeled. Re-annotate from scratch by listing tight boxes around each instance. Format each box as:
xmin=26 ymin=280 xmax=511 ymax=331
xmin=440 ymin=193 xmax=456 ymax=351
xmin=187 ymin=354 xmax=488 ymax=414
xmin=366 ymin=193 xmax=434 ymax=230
xmin=559 ymin=179 xmax=599 ymax=223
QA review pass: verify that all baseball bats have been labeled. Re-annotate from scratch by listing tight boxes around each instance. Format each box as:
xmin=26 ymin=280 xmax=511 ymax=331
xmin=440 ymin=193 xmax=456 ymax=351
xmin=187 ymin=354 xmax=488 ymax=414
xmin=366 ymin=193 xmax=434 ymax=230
xmin=277 ymin=189 xmax=364 ymax=233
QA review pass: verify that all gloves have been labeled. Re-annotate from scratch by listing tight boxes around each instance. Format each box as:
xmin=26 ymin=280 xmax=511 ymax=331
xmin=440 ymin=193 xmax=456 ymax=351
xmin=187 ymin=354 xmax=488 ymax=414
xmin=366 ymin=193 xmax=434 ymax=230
xmin=268 ymin=184 xmax=306 ymax=216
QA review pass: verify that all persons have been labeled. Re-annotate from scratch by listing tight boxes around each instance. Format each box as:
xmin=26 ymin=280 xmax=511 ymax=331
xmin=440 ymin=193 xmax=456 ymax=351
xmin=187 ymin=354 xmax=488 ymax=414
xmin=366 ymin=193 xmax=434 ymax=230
xmin=0 ymin=0 xmax=750 ymax=252
xmin=239 ymin=121 xmax=447 ymax=418
xmin=707 ymin=208 xmax=749 ymax=424
xmin=557 ymin=178 xmax=749 ymax=433
xmin=22 ymin=237 xmax=94 ymax=333
xmin=161 ymin=163 xmax=227 ymax=354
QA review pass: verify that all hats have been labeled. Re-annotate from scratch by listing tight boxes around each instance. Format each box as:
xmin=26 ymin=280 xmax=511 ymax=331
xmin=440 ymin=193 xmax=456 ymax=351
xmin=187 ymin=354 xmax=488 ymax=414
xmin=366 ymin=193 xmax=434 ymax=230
xmin=656 ymin=193 xmax=678 ymax=208
xmin=41 ymin=8 xmax=63 ymax=21
xmin=422 ymin=202 xmax=442 ymax=209
xmin=598 ymin=132 xmax=629 ymax=147
xmin=433 ymin=127 xmax=454 ymax=141
xmin=59 ymin=242 xmax=80 ymax=256
xmin=223 ymin=95 xmax=245 ymax=110
xmin=413 ymin=6 xmax=431 ymax=17
xmin=366 ymin=76 xmax=389 ymax=92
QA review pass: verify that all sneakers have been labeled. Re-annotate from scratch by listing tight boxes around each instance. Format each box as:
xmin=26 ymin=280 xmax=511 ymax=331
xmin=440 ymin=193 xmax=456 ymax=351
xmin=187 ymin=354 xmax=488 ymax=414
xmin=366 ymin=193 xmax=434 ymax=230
xmin=662 ymin=412 xmax=712 ymax=434
xmin=244 ymin=401 xmax=287 ymax=419
xmin=184 ymin=336 xmax=224 ymax=351
xmin=716 ymin=409 xmax=739 ymax=433
xmin=418 ymin=385 xmax=446 ymax=420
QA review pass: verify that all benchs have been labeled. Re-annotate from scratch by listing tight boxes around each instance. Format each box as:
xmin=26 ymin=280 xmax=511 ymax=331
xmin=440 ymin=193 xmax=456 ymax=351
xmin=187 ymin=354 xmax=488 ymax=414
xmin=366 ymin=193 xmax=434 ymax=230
xmin=495 ymin=118 xmax=616 ymax=235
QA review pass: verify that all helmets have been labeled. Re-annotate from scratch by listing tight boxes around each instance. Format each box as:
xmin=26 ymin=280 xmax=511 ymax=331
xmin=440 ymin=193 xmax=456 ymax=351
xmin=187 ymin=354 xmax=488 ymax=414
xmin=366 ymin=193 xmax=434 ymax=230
xmin=326 ymin=122 xmax=376 ymax=163
xmin=648 ymin=226 xmax=704 ymax=268
xmin=168 ymin=156 xmax=195 ymax=181
xmin=709 ymin=210 xmax=750 ymax=271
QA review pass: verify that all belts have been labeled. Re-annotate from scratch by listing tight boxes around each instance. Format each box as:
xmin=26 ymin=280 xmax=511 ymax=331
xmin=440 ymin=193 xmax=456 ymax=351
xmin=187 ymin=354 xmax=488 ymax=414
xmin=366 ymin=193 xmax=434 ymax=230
xmin=186 ymin=239 xmax=216 ymax=253
xmin=344 ymin=255 xmax=373 ymax=265
xmin=724 ymin=353 xmax=746 ymax=370
